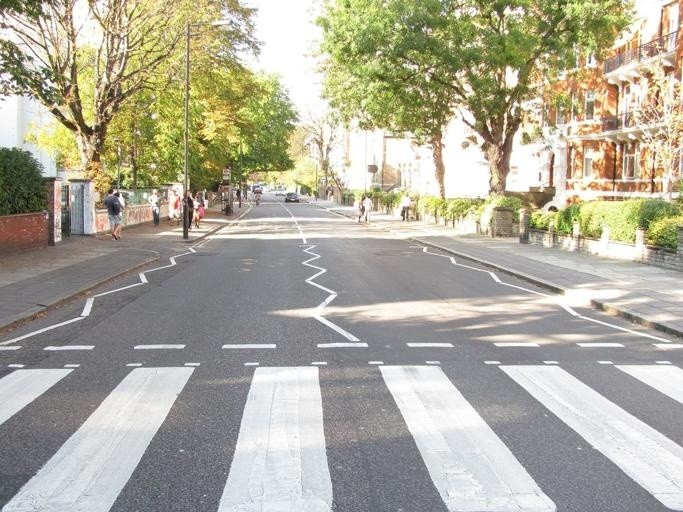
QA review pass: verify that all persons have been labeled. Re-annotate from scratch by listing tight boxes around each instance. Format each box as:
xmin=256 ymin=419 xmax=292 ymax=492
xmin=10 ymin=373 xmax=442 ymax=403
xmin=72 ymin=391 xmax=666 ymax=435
xmin=402 ymin=193 xmax=411 ymax=222
xmin=363 ymin=196 xmax=373 ymax=222
xmin=253 ymin=185 xmax=262 ymax=195
xmin=104 ymin=187 xmax=206 ymax=241
xmin=358 ymin=197 xmax=367 ymax=223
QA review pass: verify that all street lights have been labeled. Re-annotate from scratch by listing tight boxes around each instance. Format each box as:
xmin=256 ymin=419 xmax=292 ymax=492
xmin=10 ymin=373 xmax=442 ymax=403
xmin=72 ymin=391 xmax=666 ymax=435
xmin=181 ymin=18 xmax=233 ymax=241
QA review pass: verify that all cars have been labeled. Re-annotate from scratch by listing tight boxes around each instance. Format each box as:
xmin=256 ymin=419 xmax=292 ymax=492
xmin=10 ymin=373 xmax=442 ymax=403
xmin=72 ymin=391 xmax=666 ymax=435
xmin=542 ymin=190 xmax=597 ymax=214
xmin=251 ymin=185 xmax=299 ymax=202
